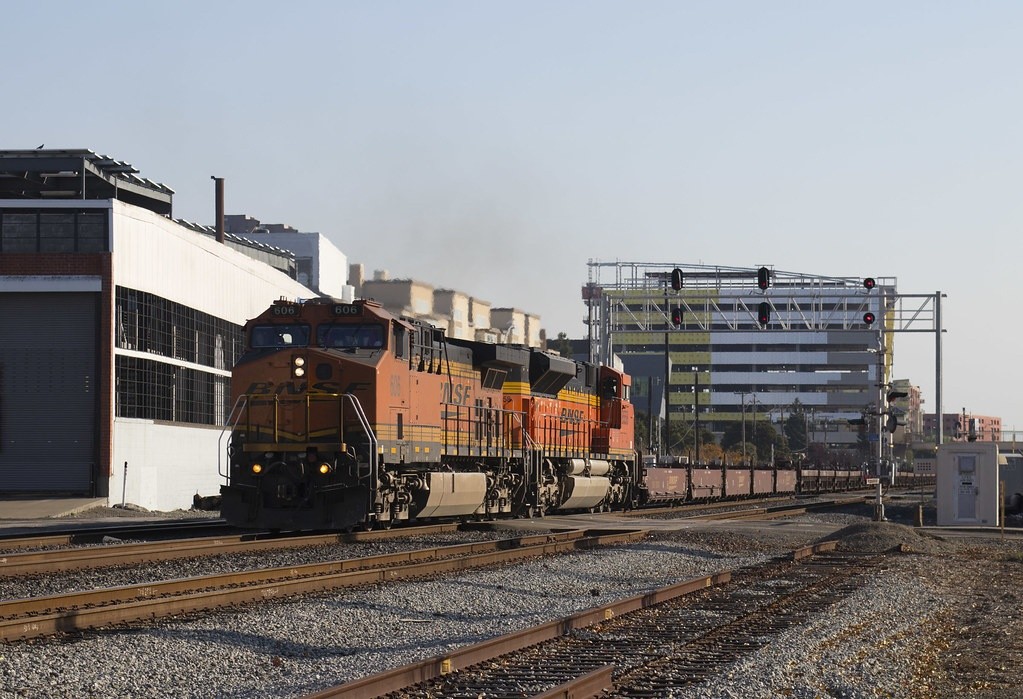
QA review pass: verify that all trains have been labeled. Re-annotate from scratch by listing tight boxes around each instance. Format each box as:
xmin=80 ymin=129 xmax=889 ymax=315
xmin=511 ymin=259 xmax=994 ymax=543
xmin=217 ymin=295 xmax=935 ymax=530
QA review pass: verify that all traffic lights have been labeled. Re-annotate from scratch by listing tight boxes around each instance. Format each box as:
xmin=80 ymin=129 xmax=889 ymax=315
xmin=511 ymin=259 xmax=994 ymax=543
xmin=672 ymin=308 xmax=683 ymax=325
xmin=759 ymin=268 xmax=770 ymax=290
xmin=671 ymin=269 xmax=683 ymax=291
xmin=863 ymin=278 xmax=875 ymax=289
xmin=758 ymin=302 xmax=771 ymax=324
xmin=863 ymin=313 xmax=876 ymax=324
